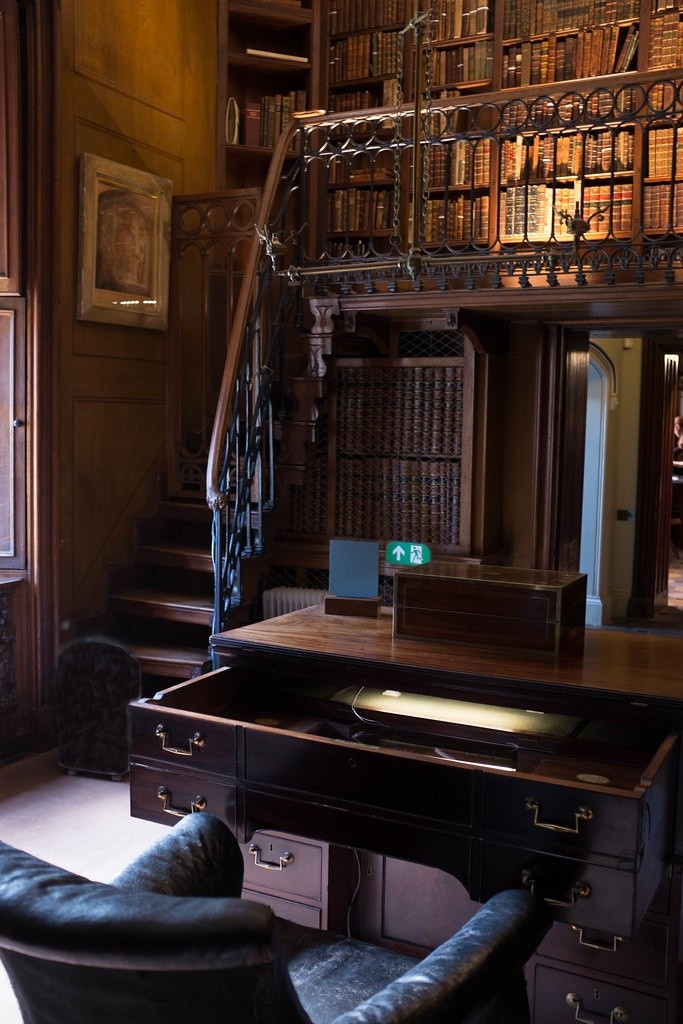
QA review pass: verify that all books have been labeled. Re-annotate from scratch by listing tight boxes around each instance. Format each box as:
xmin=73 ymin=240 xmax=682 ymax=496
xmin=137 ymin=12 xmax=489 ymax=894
xmin=287 ymin=366 xmax=469 ymax=546
xmin=240 ymin=0 xmax=683 ymax=261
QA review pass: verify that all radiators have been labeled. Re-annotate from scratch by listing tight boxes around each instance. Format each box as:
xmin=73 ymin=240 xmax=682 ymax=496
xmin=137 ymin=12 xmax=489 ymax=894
xmin=262 ymin=587 xmax=327 ymax=621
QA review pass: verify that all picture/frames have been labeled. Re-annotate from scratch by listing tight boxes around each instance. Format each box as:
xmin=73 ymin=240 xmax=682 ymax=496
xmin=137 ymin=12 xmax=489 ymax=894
xmin=78 ymin=153 xmax=173 ymax=330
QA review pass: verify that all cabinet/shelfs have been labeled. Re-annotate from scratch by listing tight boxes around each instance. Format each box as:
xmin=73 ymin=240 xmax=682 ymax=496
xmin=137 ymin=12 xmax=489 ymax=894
xmin=216 ymin=0 xmax=683 ymax=279
xmin=126 ymin=608 xmax=682 ymax=1024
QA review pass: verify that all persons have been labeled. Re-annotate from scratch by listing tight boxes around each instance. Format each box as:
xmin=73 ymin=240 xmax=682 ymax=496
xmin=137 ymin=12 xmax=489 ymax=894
xmin=673 ymin=417 xmax=683 ymax=462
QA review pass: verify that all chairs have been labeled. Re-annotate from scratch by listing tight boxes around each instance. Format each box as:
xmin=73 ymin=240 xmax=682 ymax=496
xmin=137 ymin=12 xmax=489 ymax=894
xmin=0 ymin=812 xmax=552 ymax=1024
